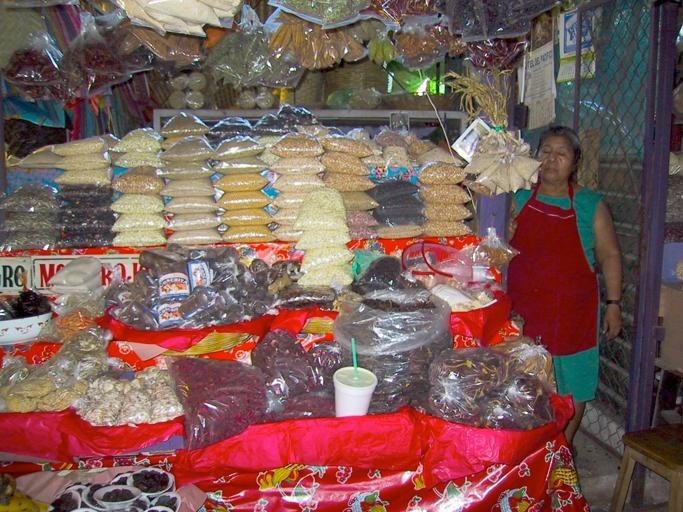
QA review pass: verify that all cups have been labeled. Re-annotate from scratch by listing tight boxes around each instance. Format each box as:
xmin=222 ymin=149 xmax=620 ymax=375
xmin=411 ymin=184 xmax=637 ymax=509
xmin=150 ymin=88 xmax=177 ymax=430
xmin=333 ymin=364 xmax=378 ymax=417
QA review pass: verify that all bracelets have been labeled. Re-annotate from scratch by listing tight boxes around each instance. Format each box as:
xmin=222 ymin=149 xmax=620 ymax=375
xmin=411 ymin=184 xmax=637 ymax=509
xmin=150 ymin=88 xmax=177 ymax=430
xmin=606 ymin=300 xmax=620 ymax=304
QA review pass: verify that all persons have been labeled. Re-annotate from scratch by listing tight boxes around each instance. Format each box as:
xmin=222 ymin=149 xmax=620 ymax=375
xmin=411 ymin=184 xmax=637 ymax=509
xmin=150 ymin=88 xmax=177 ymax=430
xmin=509 ymin=126 xmax=623 ymax=457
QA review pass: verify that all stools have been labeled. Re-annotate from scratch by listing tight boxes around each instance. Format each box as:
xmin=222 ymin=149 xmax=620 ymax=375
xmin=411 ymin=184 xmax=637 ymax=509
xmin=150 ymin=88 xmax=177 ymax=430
xmin=609 ymin=423 xmax=683 ymax=511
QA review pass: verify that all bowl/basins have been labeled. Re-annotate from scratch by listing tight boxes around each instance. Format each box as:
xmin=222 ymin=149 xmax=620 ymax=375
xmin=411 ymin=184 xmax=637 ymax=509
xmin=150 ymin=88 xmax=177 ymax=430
xmin=47 ymin=467 xmax=180 ymax=511
